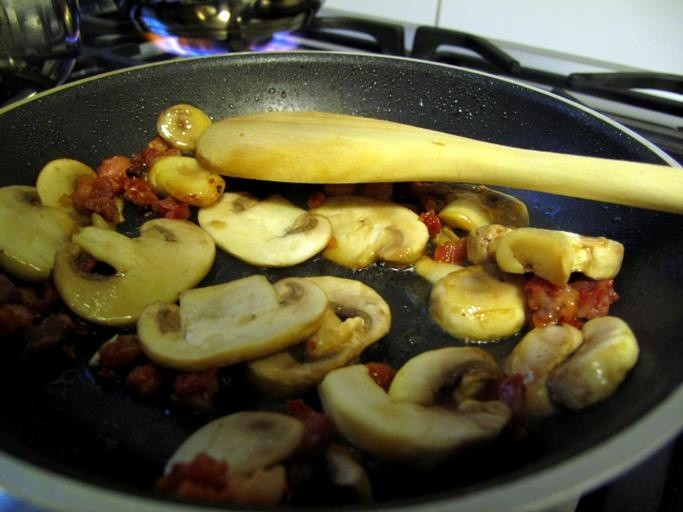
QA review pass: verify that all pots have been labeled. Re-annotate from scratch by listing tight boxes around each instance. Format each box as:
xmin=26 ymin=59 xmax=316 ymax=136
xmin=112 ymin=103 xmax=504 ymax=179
xmin=0 ymin=47 xmax=683 ymax=511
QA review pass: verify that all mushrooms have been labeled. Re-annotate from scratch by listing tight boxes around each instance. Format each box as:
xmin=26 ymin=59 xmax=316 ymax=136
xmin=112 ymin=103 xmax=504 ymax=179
xmin=0 ymin=103 xmax=639 ymax=506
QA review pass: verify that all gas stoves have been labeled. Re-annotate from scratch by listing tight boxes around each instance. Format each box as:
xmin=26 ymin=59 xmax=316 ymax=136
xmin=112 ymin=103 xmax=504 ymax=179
xmin=0 ymin=7 xmax=683 ymax=167
